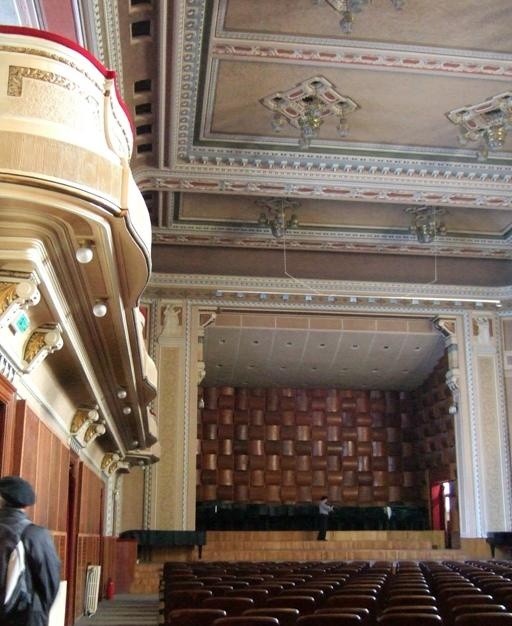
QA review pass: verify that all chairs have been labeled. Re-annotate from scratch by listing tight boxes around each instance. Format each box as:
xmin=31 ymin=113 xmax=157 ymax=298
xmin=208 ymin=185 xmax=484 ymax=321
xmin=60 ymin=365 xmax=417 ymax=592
xmin=158 ymin=559 xmax=512 ymax=626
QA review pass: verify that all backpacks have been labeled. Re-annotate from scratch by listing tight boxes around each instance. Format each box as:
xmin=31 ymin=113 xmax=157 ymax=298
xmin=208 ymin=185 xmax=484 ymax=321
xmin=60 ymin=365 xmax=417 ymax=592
xmin=0 ymin=518 xmax=37 ymax=626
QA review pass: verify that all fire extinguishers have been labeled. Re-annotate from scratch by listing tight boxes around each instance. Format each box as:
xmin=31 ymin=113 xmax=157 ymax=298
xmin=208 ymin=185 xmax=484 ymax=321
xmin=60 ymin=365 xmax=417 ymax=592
xmin=107 ymin=577 xmax=114 ymax=600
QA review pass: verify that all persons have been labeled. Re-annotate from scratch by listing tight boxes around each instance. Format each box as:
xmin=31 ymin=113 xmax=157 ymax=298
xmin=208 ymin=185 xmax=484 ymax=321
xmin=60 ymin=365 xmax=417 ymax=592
xmin=316 ymin=495 xmax=335 ymax=541
xmin=1 ymin=476 xmax=61 ymax=626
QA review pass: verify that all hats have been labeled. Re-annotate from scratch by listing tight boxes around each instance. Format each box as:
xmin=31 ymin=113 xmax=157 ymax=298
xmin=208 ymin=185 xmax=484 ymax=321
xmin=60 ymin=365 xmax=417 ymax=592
xmin=0 ymin=475 xmax=36 ymax=509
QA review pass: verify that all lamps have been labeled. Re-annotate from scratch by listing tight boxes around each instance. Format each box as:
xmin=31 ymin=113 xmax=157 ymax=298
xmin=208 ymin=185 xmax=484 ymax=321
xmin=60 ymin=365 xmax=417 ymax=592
xmin=270 ymin=1 xmax=512 ymax=150
xmin=75 ymin=239 xmax=132 ymax=416
